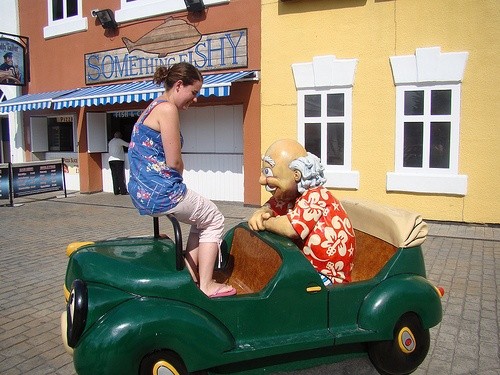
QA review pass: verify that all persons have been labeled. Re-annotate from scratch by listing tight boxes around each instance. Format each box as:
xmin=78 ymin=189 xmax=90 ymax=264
xmin=247 ymin=137 xmax=355 ymax=287
xmin=108 ymin=131 xmax=131 ymax=195
xmin=127 ymin=63 xmax=237 ymax=297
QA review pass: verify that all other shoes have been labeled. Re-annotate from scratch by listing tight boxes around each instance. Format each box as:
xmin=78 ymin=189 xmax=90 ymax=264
xmin=122 ymin=192 xmax=129 ymax=195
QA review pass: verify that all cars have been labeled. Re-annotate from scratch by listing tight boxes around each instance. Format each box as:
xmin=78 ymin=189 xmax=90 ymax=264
xmin=58 ymin=195 xmax=445 ymax=372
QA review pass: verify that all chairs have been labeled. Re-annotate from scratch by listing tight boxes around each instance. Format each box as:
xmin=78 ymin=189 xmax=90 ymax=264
xmin=198 ymin=224 xmax=283 ymax=295
xmin=340 ymin=201 xmax=402 ymax=283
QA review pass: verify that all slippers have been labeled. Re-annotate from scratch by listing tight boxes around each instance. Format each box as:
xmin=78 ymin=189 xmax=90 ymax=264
xmin=208 ymin=284 xmax=236 ymax=298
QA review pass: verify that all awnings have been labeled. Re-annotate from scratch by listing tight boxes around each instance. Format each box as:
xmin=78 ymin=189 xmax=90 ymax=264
xmin=0 ymin=71 xmax=255 ymax=113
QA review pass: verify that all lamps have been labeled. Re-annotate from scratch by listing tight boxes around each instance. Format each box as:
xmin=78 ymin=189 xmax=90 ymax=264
xmin=184 ymin=0 xmax=205 ymax=12
xmin=95 ymin=9 xmax=118 ymax=29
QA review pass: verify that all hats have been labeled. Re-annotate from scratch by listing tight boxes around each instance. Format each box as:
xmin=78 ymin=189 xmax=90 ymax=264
xmin=3 ymin=52 xmax=13 ymax=57
